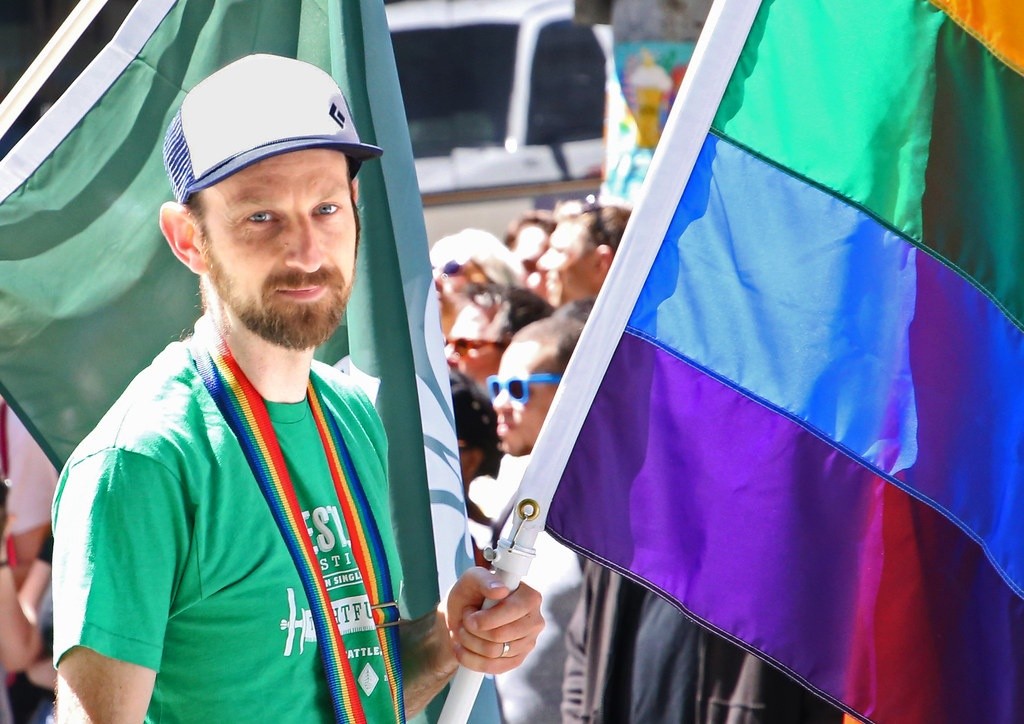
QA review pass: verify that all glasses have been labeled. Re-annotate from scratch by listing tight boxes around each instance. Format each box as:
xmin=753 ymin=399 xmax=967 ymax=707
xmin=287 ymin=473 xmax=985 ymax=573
xmin=444 ymin=336 xmax=510 ymax=358
xmin=550 ymin=196 xmax=606 ymax=223
xmin=434 ymin=256 xmax=484 ymax=289
xmin=486 ymin=372 xmax=563 ymax=404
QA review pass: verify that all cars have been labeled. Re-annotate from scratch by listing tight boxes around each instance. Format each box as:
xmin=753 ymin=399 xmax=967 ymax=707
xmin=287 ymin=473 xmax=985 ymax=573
xmin=375 ymin=0 xmax=640 ymax=195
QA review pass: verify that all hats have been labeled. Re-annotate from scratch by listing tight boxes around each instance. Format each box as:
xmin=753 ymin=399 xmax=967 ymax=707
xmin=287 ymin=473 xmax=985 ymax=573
xmin=165 ymin=55 xmax=383 ymax=204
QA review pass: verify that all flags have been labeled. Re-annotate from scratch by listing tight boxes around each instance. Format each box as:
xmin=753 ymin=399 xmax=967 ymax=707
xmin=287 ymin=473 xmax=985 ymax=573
xmin=1 ymin=0 xmax=508 ymax=724
xmin=538 ymin=0 xmax=1023 ymax=724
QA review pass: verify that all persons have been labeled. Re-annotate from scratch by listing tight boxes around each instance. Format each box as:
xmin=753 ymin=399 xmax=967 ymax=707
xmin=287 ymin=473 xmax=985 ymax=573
xmin=429 ymin=200 xmax=847 ymax=724
xmin=0 ymin=481 xmax=58 ymax=724
xmin=52 ymin=54 xmax=546 ymax=724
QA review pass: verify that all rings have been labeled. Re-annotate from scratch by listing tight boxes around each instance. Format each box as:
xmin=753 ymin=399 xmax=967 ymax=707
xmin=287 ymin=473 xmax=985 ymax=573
xmin=498 ymin=642 xmax=510 ymax=658
